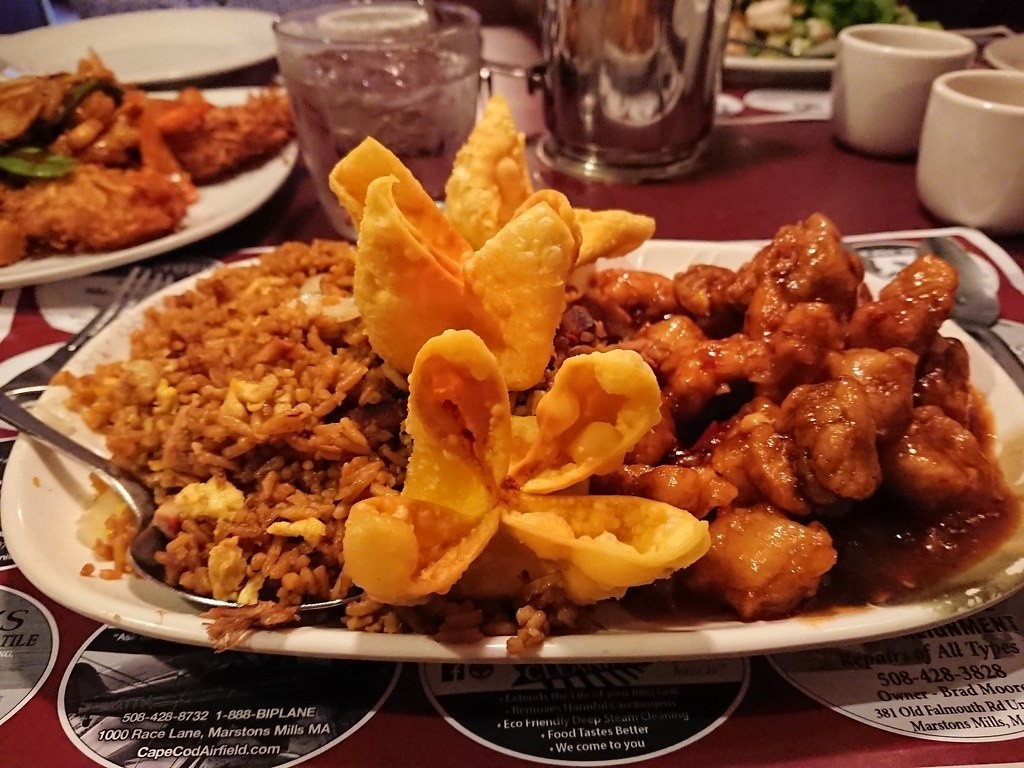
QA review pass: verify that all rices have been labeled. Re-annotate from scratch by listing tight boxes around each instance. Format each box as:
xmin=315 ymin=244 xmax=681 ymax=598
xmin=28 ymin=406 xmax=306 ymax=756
xmin=49 ymin=237 xmax=585 ymax=654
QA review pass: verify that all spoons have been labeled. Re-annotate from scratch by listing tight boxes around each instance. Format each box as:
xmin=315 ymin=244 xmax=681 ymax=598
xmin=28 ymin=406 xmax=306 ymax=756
xmin=916 ymin=235 xmax=1024 ymax=396
xmin=1 ymin=391 xmax=349 ymax=610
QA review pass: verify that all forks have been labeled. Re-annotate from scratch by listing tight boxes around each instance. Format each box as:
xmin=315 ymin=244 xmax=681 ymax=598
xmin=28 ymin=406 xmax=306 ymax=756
xmin=0 ymin=266 xmax=189 ymax=392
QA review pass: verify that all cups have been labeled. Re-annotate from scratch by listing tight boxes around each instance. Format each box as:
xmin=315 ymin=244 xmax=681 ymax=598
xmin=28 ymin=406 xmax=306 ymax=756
xmin=832 ymin=25 xmax=978 ymax=154
xmin=272 ymin=0 xmax=483 ymax=240
xmin=530 ymin=1 xmax=730 ymax=173
xmin=915 ymin=67 xmax=1024 ymax=233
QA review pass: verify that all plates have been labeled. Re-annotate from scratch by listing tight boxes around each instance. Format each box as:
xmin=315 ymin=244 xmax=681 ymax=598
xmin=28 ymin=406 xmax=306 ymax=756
xmin=1 ymin=8 xmax=278 ymax=88
xmin=982 ymin=34 xmax=1024 ymax=69
xmin=0 ymin=86 xmax=299 ymax=285
xmin=1 ymin=239 xmax=1024 ymax=665
xmin=722 ymin=55 xmax=834 ymax=87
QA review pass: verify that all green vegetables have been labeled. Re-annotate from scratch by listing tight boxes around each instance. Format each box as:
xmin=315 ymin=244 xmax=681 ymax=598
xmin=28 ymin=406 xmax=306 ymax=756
xmin=750 ymin=0 xmax=906 ymax=57
xmin=0 ymin=78 xmax=123 ymax=179
